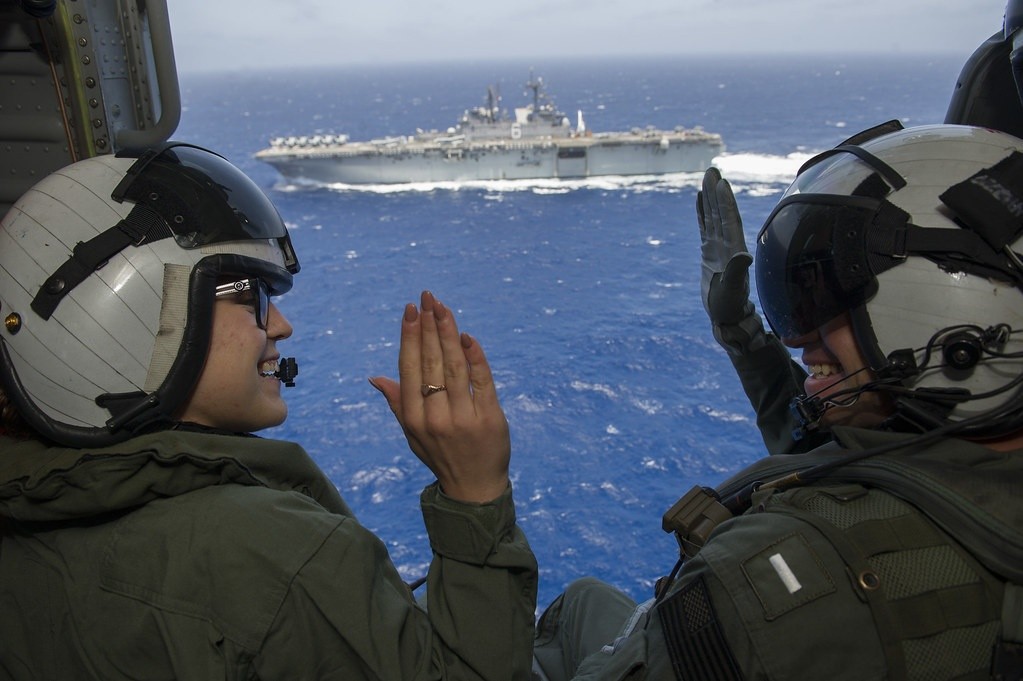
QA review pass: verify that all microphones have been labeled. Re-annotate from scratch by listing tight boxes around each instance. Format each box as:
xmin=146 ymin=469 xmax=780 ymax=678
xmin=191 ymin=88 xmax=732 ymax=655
xmin=276 ymin=357 xmax=298 ymax=387
xmin=788 ymin=324 xmax=1009 ymax=441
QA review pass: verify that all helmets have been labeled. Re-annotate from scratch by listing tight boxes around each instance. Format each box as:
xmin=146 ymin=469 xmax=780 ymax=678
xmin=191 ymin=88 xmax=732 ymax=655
xmin=0 ymin=153 xmax=293 ymax=446
xmin=773 ymin=124 xmax=1023 ymax=441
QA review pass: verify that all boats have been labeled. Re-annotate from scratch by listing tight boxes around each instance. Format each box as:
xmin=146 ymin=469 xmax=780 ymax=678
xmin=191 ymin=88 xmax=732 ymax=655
xmin=253 ymin=63 xmax=728 ymax=184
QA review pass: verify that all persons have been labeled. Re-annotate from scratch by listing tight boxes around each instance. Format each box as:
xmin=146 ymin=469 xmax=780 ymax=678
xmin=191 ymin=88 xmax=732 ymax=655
xmin=0 ymin=141 xmax=539 ymax=681
xmin=531 ymin=118 xmax=1023 ymax=681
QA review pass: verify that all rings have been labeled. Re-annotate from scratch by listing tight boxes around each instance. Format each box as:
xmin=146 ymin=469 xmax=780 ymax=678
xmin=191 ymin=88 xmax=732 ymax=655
xmin=421 ymin=384 xmax=447 ymax=397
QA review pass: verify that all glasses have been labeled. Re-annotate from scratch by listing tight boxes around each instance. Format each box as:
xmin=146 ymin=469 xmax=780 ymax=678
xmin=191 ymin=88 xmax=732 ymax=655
xmin=111 ymin=141 xmax=301 ymax=273
xmin=215 ymin=277 xmax=271 ymax=330
xmin=755 ymin=192 xmax=890 ymax=346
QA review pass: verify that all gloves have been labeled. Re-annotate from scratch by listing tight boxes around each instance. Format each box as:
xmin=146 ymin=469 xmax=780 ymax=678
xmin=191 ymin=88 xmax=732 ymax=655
xmin=696 ymin=166 xmax=769 ymax=355
